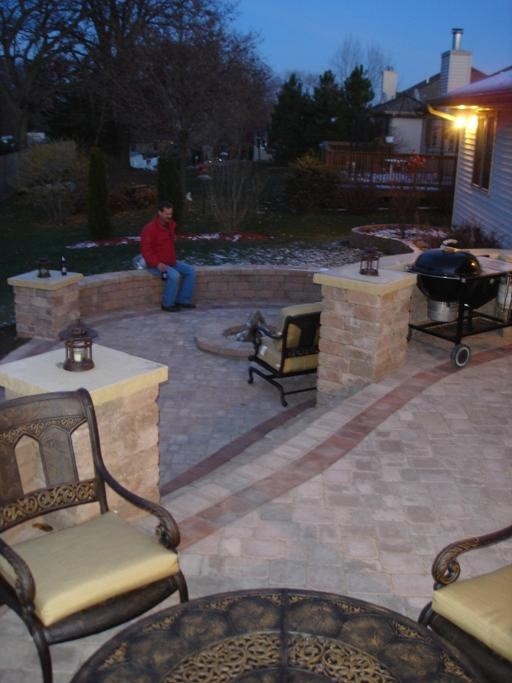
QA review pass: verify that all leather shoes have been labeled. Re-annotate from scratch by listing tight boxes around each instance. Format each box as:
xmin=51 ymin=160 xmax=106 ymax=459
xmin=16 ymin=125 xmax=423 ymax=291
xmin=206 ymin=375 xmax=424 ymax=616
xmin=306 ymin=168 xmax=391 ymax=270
xmin=161 ymin=303 xmax=196 ymax=311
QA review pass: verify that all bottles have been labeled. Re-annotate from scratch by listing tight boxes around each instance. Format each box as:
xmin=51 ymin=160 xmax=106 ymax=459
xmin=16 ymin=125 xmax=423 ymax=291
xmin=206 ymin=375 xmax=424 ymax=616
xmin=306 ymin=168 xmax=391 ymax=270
xmin=162 ymin=270 xmax=167 ymax=280
xmin=60 ymin=256 xmax=67 ymax=274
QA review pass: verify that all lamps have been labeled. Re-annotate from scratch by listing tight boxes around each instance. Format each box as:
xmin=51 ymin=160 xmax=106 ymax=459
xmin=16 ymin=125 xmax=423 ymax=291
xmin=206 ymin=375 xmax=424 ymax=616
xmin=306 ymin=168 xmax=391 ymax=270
xmin=38 ymin=256 xmax=52 ymax=278
xmin=59 ymin=319 xmax=98 ymax=370
xmin=359 ymin=245 xmax=382 ymax=276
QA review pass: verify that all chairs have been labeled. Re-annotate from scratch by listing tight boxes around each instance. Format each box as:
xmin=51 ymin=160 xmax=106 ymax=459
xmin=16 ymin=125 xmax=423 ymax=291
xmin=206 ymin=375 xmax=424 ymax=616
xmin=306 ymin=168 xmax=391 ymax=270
xmin=247 ymin=302 xmax=321 ymax=408
xmin=0 ymin=388 xmax=188 ymax=683
xmin=418 ymin=525 xmax=512 ymax=683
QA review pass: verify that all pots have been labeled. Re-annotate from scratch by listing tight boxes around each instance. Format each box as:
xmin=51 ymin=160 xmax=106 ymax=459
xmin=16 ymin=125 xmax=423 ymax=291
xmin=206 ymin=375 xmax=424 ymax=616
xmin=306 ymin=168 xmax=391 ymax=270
xmin=413 ymin=246 xmax=483 ymax=302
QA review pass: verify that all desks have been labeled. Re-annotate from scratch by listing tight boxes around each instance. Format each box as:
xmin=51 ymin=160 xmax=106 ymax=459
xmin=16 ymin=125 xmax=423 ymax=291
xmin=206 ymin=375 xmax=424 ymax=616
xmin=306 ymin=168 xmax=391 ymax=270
xmin=70 ymin=586 xmax=485 ymax=682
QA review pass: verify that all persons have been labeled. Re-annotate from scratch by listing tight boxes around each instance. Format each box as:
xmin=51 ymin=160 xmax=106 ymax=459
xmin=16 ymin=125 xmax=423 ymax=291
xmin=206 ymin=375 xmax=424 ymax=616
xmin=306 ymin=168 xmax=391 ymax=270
xmin=138 ymin=202 xmax=197 ymax=312
